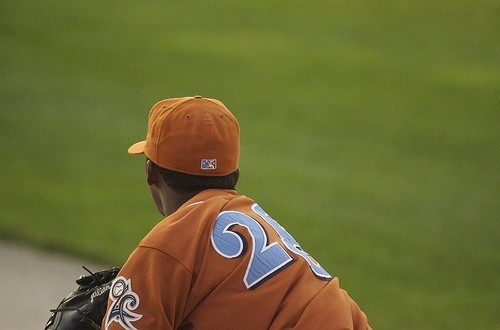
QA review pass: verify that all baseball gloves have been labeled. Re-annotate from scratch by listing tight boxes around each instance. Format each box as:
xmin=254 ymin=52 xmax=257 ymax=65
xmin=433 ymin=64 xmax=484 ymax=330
xmin=44 ymin=265 xmax=122 ymax=330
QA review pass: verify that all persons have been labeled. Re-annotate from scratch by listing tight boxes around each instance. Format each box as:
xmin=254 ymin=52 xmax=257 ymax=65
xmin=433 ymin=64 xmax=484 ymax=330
xmin=100 ymin=95 xmax=373 ymax=330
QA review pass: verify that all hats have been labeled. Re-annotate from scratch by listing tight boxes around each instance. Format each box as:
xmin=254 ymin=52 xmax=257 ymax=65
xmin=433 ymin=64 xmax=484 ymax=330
xmin=127 ymin=96 xmax=240 ymax=176
